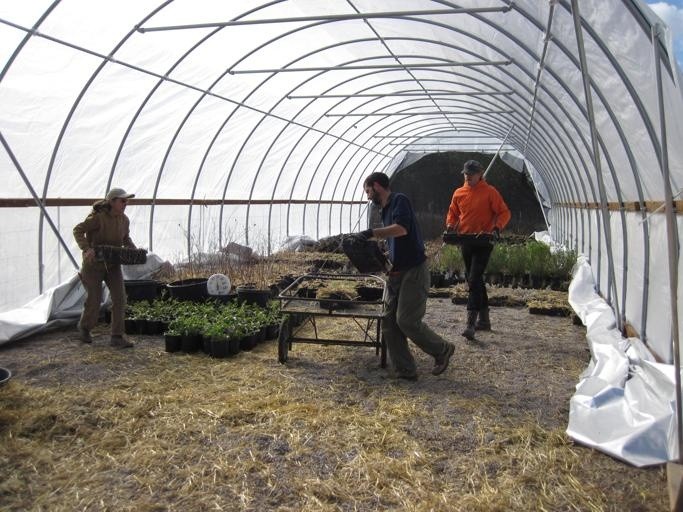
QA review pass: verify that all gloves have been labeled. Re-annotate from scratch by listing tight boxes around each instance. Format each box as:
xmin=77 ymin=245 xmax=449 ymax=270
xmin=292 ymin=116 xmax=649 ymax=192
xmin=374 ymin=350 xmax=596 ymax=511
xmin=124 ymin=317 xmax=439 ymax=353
xmin=446 ymin=223 xmax=454 ymax=234
xmin=489 ymin=225 xmax=502 ymax=242
xmin=352 ymin=229 xmax=374 ymax=247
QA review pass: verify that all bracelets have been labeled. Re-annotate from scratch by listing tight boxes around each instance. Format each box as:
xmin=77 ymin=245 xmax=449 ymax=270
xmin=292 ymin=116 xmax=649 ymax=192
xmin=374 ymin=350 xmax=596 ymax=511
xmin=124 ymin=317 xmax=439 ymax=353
xmin=84 ymin=248 xmax=89 ymax=253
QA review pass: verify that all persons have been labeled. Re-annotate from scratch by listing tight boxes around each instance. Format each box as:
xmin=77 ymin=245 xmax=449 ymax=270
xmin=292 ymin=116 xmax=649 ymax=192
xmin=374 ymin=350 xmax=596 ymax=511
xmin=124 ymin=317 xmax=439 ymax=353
xmin=73 ymin=188 xmax=138 ymax=348
xmin=446 ymin=160 xmax=511 ymax=340
xmin=357 ymin=172 xmax=455 ymax=382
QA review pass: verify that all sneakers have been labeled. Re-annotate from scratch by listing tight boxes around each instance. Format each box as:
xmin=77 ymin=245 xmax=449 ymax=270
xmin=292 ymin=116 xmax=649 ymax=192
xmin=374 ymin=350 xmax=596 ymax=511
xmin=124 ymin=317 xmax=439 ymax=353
xmin=396 ymin=368 xmax=418 ymax=382
xmin=78 ymin=321 xmax=92 ymax=343
xmin=111 ymin=335 xmax=136 ymax=347
xmin=431 ymin=342 xmax=455 ymax=375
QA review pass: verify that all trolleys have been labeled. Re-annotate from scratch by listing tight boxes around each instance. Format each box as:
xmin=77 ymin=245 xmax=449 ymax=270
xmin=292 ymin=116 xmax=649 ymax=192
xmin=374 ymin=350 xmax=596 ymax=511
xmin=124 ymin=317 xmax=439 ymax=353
xmin=275 ymin=272 xmax=391 ymax=370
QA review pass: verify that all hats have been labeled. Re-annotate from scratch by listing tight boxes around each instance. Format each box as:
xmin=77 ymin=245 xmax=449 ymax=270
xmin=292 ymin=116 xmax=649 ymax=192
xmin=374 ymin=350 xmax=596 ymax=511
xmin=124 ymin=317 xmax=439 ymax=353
xmin=461 ymin=160 xmax=484 ymax=174
xmin=107 ymin=189 xmax=135 ymax=201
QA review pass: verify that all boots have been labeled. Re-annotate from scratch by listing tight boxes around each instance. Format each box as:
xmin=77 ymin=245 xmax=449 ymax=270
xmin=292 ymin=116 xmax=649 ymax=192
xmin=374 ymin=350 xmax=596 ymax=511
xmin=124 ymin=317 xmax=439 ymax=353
xmin=476 ymin=308 xmax=491 ymax=330
xmin=462 ymin=310 xmax=477 ymax=340
